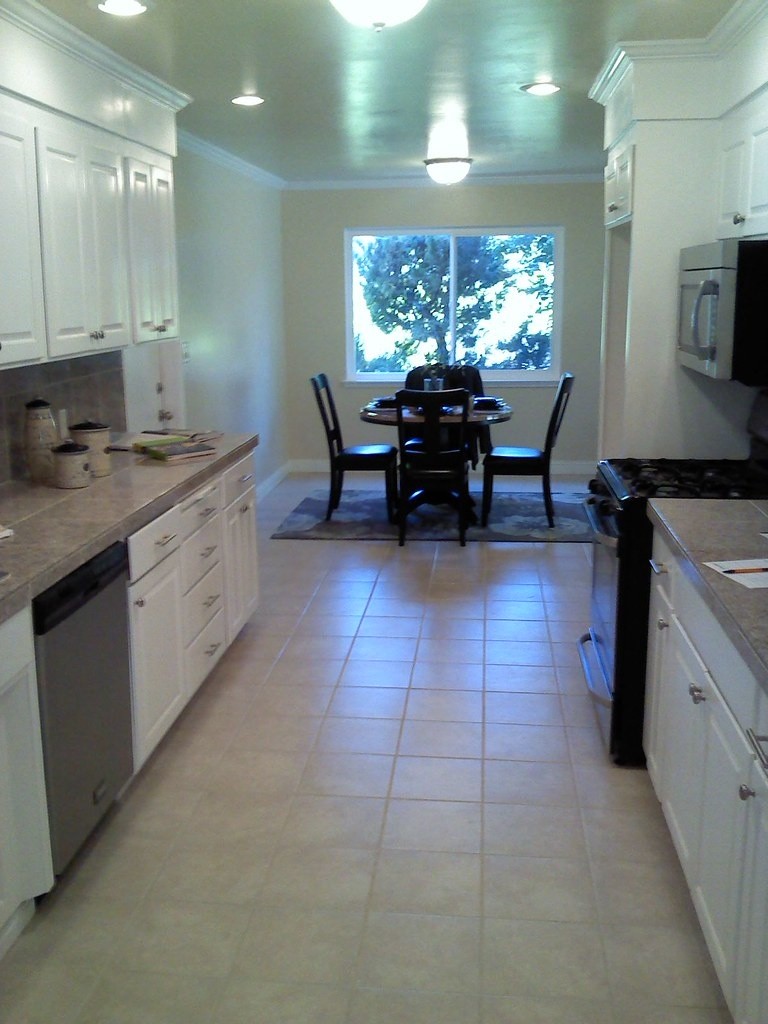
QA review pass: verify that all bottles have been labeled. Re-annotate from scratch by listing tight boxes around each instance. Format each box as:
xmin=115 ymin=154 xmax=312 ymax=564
xmin=25 ymin=398 xmax=56 ymax=481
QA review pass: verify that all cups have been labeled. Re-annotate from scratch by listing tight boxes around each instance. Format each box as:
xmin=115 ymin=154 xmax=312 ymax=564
xmin=51 ymin=438 xmax=89 ymax=488
xmin=68 ymin=418 xmax=111 ymax=478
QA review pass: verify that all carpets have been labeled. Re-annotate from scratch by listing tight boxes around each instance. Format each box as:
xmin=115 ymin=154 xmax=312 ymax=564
xmin=271 ymin=488 xmax=599 ymax=543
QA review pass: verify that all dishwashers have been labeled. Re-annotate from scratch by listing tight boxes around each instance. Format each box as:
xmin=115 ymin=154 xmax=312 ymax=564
xmin=32 ymin=541 xmax=134 ymax=875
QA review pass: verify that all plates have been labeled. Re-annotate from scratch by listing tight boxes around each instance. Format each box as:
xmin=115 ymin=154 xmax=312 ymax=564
xmin=474 ymin=411 xmax=502 ymax=413
xmin=366 ymin=407 xmax=397 ymax=411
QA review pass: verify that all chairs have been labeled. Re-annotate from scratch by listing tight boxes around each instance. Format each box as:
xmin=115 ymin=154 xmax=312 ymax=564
xmin=394 ymin=363 xmax=488 ymax=547
xmin=310 ymin=374 xmax=397 ymax=524
xmin=481 ymin=373 xmax=575 ymax=529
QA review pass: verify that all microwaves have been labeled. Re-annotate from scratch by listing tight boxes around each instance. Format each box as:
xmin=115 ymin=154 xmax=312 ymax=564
xmin=675 ymin=239 xmax=768 ymax=386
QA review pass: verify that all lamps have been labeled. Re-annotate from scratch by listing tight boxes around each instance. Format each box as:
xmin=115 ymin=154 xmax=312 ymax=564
xmin=330 ymin=0 xmax=429 ymax=32
xmin=424 ymin=158 xmax=472 ymax=185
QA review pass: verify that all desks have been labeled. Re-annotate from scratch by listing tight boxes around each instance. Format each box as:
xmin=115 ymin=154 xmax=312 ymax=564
xmin=360 ymin=405 xmax=512 ymax=532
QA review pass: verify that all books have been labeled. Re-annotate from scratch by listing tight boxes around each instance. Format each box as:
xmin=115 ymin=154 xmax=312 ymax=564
xmin=134 ymin=434 xmax=218 ymax=461
xmin=474 ymin=410 xmax=501 ymax=414
xmin=366 ymin=405 xmax=396 ymax=412
xmin=106 ymin=428 xmax=213 ymax=450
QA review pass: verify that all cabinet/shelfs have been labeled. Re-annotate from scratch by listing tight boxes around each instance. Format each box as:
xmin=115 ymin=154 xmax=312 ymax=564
xmin=0 ymin=0 xmax=193 ymax=366
xmin=603 ymin=144 xmax=635 ymax=226
xmin=123 ymin=448 xmax=262 ymax=788
xmin=0 ymin=601 xmax=55 ymax=967
xmin=715 ymin=107 xmax=768 ymax=243
xmin=645 ymin=525 xmax=768 ymax=1024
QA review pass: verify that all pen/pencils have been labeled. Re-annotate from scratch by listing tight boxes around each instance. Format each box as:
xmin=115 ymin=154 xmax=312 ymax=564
xmin=722 ymin=567 xmax=768 ymax=574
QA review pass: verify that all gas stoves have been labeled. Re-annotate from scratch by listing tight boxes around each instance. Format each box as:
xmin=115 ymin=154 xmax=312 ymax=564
xmin=597 ymin=436 xmax=768 ymax=504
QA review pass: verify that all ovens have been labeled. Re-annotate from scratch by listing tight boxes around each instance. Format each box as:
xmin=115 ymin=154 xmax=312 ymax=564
xmin=577 ymin=468 xmax=653 ymax=767
